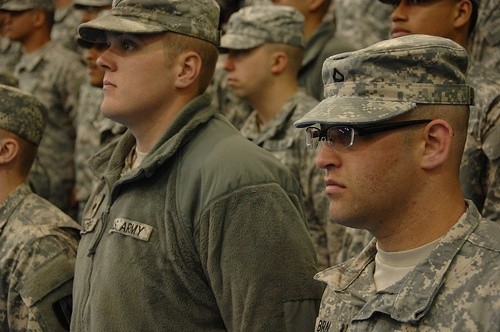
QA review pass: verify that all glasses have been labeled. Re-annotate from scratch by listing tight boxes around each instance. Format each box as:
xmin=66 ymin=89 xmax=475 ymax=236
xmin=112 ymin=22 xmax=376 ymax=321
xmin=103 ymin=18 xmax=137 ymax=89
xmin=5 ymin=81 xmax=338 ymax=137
xmin=305 ymin=119 xmax=433 ymax=152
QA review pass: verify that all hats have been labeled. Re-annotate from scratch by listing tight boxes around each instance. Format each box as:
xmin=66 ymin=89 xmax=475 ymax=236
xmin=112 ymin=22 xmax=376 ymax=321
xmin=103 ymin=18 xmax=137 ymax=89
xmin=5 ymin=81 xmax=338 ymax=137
xmin=220 ymin=6 xmax=304 ymax=49
xmin=294 ymin=35 xmax=475 ymax=129
xmin=78 ymin=0 xmax=220 ymax=46
xmin=0 ymin=83 xmax=48 ymax=147
xmin=0 ymin=0 xmax=54 ymax=11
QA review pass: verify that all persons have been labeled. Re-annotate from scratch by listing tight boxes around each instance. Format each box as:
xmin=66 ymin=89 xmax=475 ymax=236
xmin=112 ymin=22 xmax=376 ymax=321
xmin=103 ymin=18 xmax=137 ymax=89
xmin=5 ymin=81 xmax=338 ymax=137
xmin=0 ymin=84 xmax=83 ymax=332
xmin=0 ymin=1 xmax=500 ymax=268
xmin=293 ymin=35 xmax=500 ymax=332
xmin=69 ymin=1 xmax=327 ymax=332
